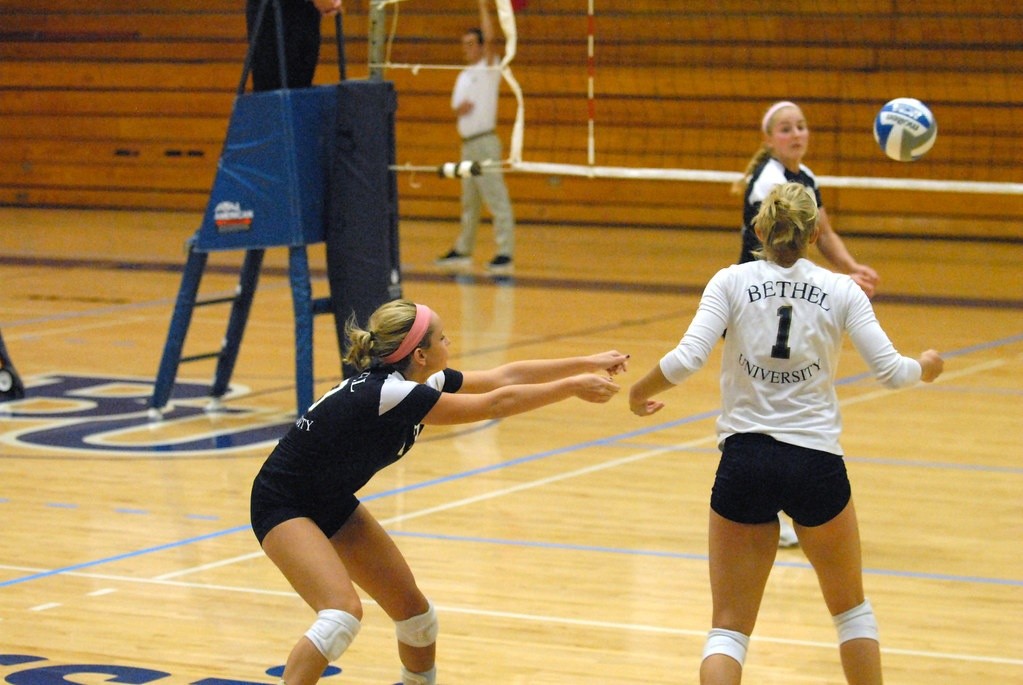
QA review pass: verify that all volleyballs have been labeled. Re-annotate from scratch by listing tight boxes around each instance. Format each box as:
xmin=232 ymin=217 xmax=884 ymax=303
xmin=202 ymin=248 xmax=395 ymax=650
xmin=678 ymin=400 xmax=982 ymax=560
xmin=872 ymin=97 xmax=938 ymax=162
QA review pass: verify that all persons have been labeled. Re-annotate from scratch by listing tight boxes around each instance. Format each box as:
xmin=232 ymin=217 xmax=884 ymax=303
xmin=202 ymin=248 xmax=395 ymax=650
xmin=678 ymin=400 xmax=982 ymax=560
xmin=723 ymin=99 xmax=882 ymax=547
xmin=244 ymin=0 xmax=345 ymax=92
xmin=251 ymin=300 xmax=631 ymax=685
xmin=631 ymin=182 xmax=946 ymax=685
xmin=438 ymin=0 xmax=517 ymax=267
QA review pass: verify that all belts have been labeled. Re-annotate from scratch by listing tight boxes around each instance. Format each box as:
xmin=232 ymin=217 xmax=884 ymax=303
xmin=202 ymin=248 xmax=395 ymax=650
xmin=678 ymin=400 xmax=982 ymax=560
xmin=464 ymin=130 xmax=494 ymax=143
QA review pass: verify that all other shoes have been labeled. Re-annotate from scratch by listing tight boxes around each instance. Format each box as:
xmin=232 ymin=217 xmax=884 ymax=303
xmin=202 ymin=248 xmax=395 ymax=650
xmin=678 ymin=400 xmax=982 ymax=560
xmin=778 ymin=515 xmax=799 ymax=547
xmin=437 ymin=249 xmax=472 ymax=265
xmin=490 ymin=255 xmax=512 ymax=265
